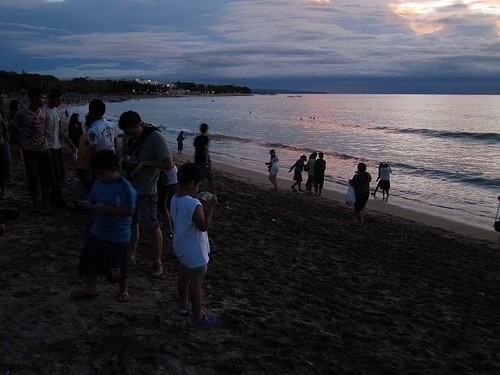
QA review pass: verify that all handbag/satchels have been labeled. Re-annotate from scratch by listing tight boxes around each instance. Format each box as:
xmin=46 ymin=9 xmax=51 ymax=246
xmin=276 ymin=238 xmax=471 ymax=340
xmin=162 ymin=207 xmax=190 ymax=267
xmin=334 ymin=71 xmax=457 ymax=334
xmin=493 ymin=221 xmax=500 ymax=232
xmin=304 ymin=165 xmax=310 ymax=172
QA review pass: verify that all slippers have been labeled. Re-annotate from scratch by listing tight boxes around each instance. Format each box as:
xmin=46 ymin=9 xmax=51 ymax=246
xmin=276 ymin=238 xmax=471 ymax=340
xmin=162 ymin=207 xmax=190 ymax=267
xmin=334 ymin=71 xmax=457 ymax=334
xmin=70 ymin=289 xmax=99 ymax=300
xmin=177 ymin=301 xmax=191 ymax=313
xmin=191 ymin=315 xmax=216 ymax=325
xmin=117 ymin=294 xmax=128 ymax=302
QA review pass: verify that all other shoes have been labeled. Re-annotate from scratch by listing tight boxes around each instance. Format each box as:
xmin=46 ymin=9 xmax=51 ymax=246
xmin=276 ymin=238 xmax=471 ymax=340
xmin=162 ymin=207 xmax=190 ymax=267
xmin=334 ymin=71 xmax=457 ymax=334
xmin=151 ymin=263 xmax=163 ymax=275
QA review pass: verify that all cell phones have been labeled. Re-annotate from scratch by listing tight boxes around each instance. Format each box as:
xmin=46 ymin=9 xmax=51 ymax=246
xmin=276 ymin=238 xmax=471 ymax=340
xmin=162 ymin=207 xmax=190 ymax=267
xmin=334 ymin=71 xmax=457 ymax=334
xmin=200 ymin=190 xmax=214 ymax=203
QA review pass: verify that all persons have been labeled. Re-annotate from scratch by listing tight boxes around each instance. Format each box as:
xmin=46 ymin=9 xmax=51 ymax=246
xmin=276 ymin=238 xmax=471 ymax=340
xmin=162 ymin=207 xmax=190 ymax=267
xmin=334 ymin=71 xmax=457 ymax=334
xmin=348 ymin=163 xmax=373 ymax=226
xmin=264 ymin=148 xmax=326 ymax=196
xmin=170 ymin=161 xmax=219 ymax=326
xmin=0 ymin=77 xmax=186 ymax=276
xmin=192 ymin=123 xmax=216 ymax=194
xmin=374 ymin=163 xmax=393 ymax=200
xmin=76 ymin=148 xmax=137 ymax=300
